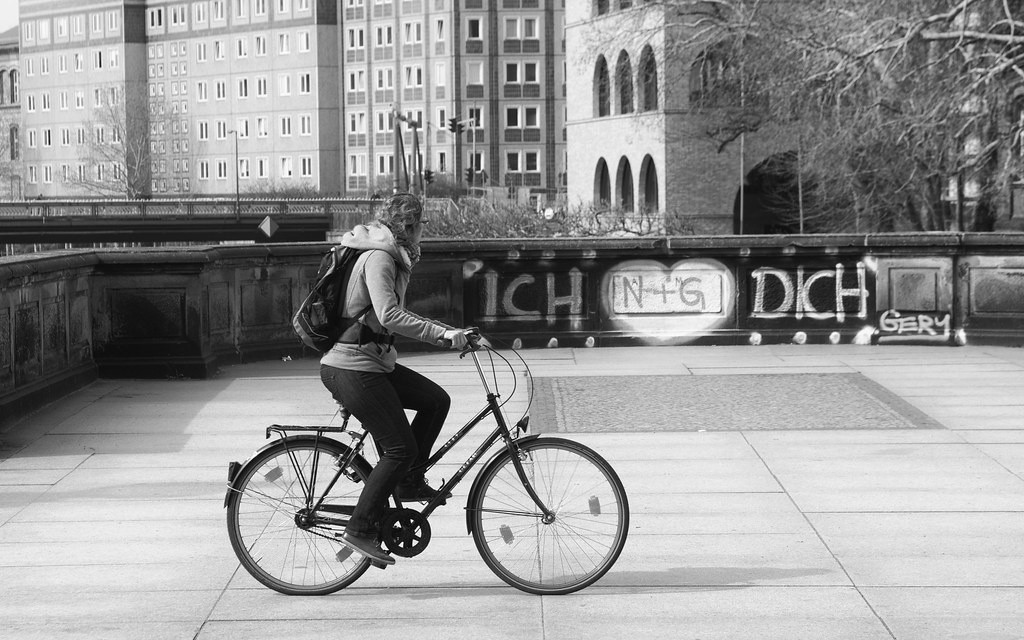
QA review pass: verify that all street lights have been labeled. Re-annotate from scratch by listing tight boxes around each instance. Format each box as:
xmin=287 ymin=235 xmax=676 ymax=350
xmin=228 ymin=130 xmax=240 ymax=223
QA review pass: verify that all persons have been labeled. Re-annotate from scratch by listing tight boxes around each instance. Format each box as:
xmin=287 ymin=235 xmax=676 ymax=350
xmin=320 ymin=193 xmax=481 ymax=564
xmin=37 ymin=194 xmax=43 ymax=201
xmin=371 ymin=192 xmax=381 ymax=199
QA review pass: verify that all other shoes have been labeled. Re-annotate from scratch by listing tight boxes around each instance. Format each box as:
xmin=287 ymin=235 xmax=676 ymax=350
xmin=342 ymin=530 xmax=396 ymax=565
xmin=396 ymin=476 xmax=452 ymax=501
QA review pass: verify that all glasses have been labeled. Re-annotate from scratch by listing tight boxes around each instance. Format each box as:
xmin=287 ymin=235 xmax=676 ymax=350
xmin=417 ymin=218 xmax=429 ymax=224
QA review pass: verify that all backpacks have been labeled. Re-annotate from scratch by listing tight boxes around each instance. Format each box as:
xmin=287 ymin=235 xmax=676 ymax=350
xmin=293 ymin=246 xmax=399 ymax=352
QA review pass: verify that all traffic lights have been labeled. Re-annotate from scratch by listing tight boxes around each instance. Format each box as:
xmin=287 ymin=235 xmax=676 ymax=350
xmin=449 ymin=118 xmax=456 ymax=132
xmin=425 ymin=169 xmax=434 ymax=185
xmin=466 ymin=168 xmax=473 ymax=182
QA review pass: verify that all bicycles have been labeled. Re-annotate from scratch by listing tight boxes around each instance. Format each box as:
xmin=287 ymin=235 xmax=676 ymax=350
xmin=224 ymin=322 xmax=630 ymax=596
